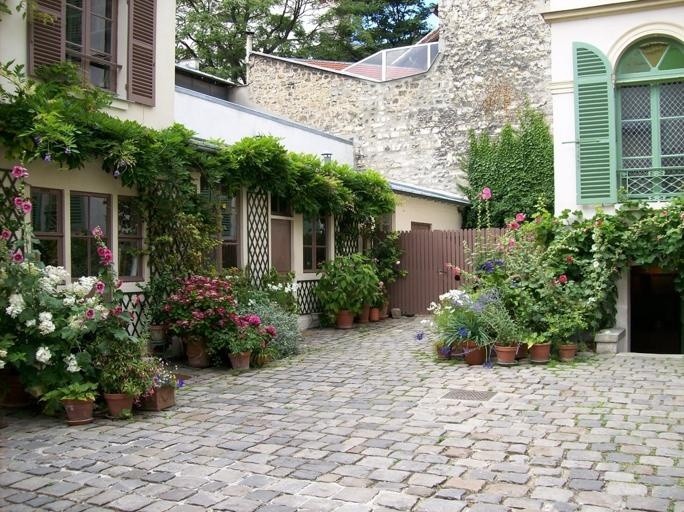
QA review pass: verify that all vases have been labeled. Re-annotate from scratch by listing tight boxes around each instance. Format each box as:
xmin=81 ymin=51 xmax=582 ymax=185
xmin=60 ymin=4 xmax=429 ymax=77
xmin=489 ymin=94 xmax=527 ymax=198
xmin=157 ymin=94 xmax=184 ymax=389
xmin=251 ymin=354 xmax=265 ymax=368
xmin=2 ymin=376 xmax=28 ymax=403
xmin=104 ymin=393 xmax=133 ymax=416
xmin=380 ymin=301 xmax=390 ymax=320
xmin=186 ymin=338 xmax=211 ymax=366
xmin=143 ymin=386 xmax=177 ymax=411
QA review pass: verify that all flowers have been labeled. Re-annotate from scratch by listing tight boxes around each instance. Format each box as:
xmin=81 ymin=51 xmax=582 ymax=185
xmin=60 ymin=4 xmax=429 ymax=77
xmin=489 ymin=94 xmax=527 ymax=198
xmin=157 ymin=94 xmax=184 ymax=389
xmin=102 ymin=348 xmax=154 ymax=407
xmin=412 ymin=257 xmax=536 ymax=368
xmin=159 ymin=271 xmax=277 ymax=353
xmin=133 ymin=355 xmax=186 ymax=406
xmin=367 ymin=230 xmax=408 ymax=308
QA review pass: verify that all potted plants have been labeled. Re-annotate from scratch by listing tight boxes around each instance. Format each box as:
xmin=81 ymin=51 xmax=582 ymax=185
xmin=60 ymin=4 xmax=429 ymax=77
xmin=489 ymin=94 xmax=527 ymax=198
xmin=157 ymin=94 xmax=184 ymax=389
xmin=40 ymin=379 xmax=101 ymax=420
xmin=136 ymin=273 xmax=181 ymax=340
xmin=209 ymin=327 xmax=273 ymax=370
xmin=544 ymin=310 xmax=589 ymax=361
xmin=312 ymin=252 xmax=379 ymax=329
xmin=524 ymin=330 xmax=554 ymax=360
